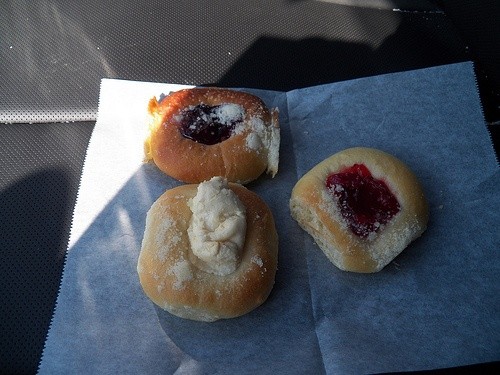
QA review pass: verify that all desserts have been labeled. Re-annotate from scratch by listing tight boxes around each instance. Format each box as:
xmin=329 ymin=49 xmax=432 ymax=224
xmin=136 ymin=176 xmax=279 ymax=322
xmin=288 ymin=147 xmax=430 ymax=273
xmin=146 ymin=88 xmax=281 ymax=183
